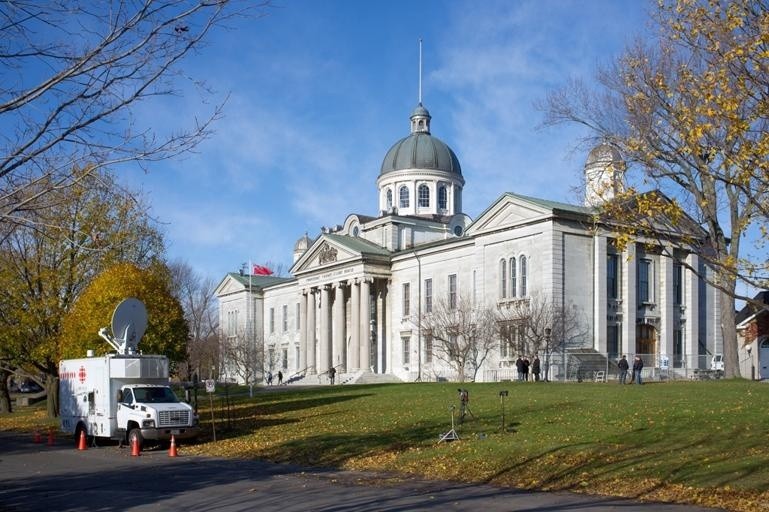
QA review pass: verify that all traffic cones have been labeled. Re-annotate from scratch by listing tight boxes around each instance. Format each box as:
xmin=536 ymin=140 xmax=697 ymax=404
xmin=166 ymin=433 xmax=180 ymax=457
xmin=127 ymin=432 xmax=142 ymax=458
xmin=32 ymin=423 xmax=43 ymax=444
xmin=46 ymin=425 xmax=55 ymax=446
xmin=75 ymin=428 xmax=90 ymax=449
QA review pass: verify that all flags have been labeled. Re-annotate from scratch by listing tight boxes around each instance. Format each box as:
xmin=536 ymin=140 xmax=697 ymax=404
xmin=253 ymin=264 xmax=273 ymax=275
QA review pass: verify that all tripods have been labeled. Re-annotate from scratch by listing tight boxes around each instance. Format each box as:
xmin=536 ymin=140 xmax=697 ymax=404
xmin=458 ymin=402 xmax=480 ymax=427
xmin=494 ymin=402 xmax=511 ymax=437
xmin=435 ymin=417 xmax=459 ymax=445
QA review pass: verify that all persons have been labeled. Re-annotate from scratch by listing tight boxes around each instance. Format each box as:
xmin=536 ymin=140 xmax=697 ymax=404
xmin=268 ymin=372 xmax=273 ymax=385
xmin=618 ymin=355 xmax=629 ymax=384
xmin=629 ymin=365 xmax=635 ymax=383
xmin=633 ymin=356 xmax=643 ymax=384
xmin=532 ymin=355 xmax=540 ymax=382
xmin=516 ymin=356 xmax=523 ymax=382
xmin=328 ymin=366 xmax=336 ymax=384
xmin=523 ymin=356 xmax=529 ymax=381
xmin=278 ymin=371 xmax=283 ymax=385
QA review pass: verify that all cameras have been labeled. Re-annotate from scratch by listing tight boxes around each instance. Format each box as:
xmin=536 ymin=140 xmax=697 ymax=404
xmin=500 ymin=390 xmax=508 ymax=396
xmin=457 ymin=388 xmax=469 ymax=401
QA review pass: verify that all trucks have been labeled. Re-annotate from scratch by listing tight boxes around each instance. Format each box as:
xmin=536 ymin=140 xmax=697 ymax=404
xmin=57 ymin=349 xmax=198 ymax=443
xmin=691 ymin=351 xmax=725 ymax=379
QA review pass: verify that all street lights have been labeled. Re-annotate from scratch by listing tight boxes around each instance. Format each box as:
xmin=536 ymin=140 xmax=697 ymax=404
xmin=542 ymin=326 xmax=553 ymax=380
